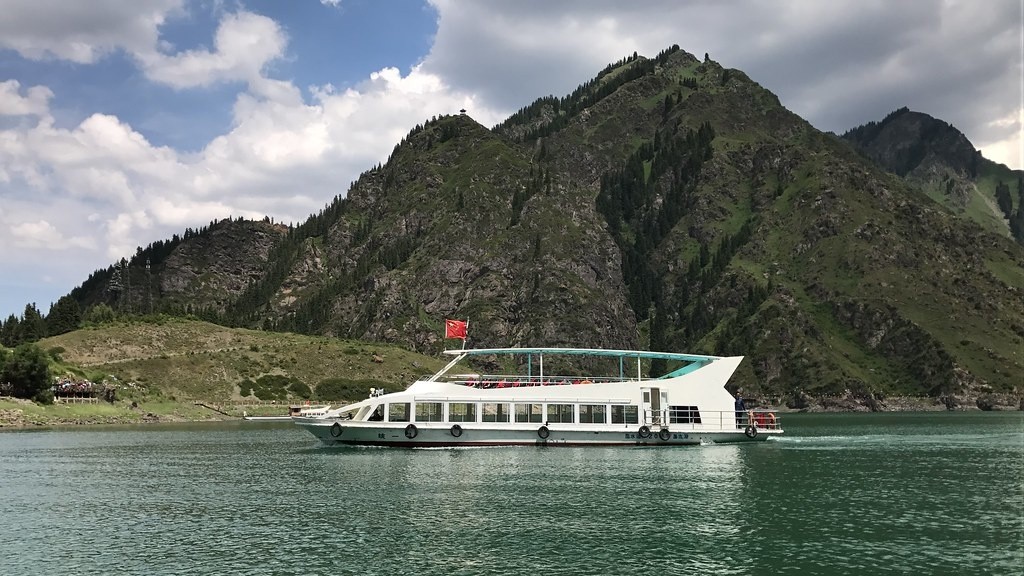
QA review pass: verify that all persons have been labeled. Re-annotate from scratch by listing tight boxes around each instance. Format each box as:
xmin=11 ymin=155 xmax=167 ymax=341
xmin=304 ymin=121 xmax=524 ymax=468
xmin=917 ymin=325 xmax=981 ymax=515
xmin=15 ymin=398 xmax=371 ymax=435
xmin=243 ymin=409 xmax=248 ymax=418
xmin=344 ymin=413 xmax=351 ymax=421
xmin=370 ymin=409 xmax=382 ymax=421
xmin=305 ymin=400 xmax=309 ymax=405
xmin=465 ymin=378 xmax=497 ymax=388
xmin=735 ymin=393 xmax=746 ymax=429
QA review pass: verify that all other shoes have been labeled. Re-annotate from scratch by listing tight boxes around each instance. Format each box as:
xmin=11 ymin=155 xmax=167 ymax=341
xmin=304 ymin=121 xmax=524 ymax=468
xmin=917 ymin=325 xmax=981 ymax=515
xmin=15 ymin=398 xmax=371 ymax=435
xmin=738 ymin=425 xmax=742 ymax=429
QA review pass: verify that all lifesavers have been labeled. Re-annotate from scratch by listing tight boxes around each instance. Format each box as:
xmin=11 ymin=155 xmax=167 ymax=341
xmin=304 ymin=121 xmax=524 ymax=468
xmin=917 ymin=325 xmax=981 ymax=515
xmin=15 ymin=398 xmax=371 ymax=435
xmin=659 ymin=429 xmax=670 ymax=441
xmin=767 ymin=413 xmax=775 ymax=429
xmin=745 ymin=425 xmax=757 ymax=438
xmin=405 ymin=424 xmax=419 ymax=439
xmin=450 ymin=424 xmax=463 ymax=438
xmin=329 ymin=423 xmax=343 ymax=437
xmin=639 ymin=425 xmax=650 ymax=438
xmin=537 ymin=426 xmax=550 ymax=439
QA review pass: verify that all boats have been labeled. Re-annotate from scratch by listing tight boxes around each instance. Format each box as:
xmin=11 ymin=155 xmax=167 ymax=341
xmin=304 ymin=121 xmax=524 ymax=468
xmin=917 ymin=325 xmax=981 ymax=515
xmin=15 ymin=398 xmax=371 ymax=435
xmin=289 ymin=314 xmax=784 ymax=445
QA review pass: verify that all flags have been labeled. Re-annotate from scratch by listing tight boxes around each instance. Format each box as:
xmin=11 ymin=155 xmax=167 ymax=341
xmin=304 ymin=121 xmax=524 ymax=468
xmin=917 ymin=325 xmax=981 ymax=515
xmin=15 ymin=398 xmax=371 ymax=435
xmin=446 ymin=320 xmax=466 ymax=338
